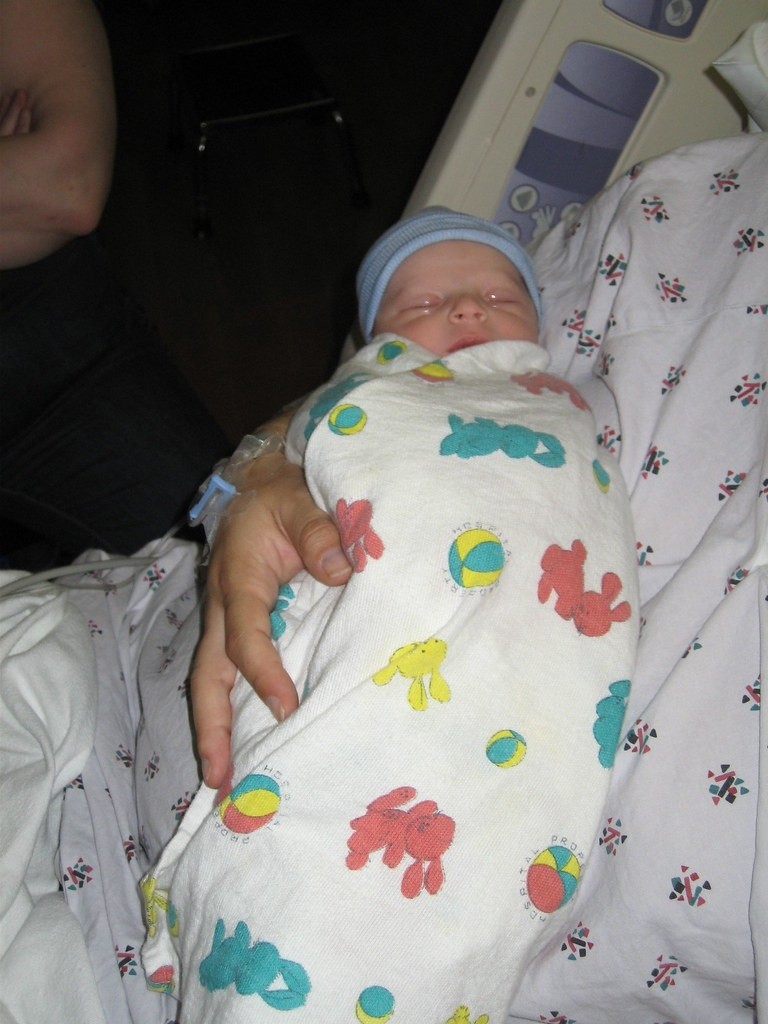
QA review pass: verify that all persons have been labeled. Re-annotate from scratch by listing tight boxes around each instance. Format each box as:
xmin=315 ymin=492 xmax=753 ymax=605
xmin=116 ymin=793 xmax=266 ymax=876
xmin=1 ymin=128 xmax=768 ymax=1024
xmin=0 ymin=1 xmax=234 ymax=577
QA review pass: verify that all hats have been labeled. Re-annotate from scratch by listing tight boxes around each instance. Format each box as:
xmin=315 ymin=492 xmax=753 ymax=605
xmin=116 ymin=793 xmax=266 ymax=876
xmin=356 ymin=204 xmax=543 ymax=348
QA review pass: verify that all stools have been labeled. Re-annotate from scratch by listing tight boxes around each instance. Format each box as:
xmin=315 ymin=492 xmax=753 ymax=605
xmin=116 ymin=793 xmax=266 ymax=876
xmin=166 ymin=32 xmax=371 ymax=243
